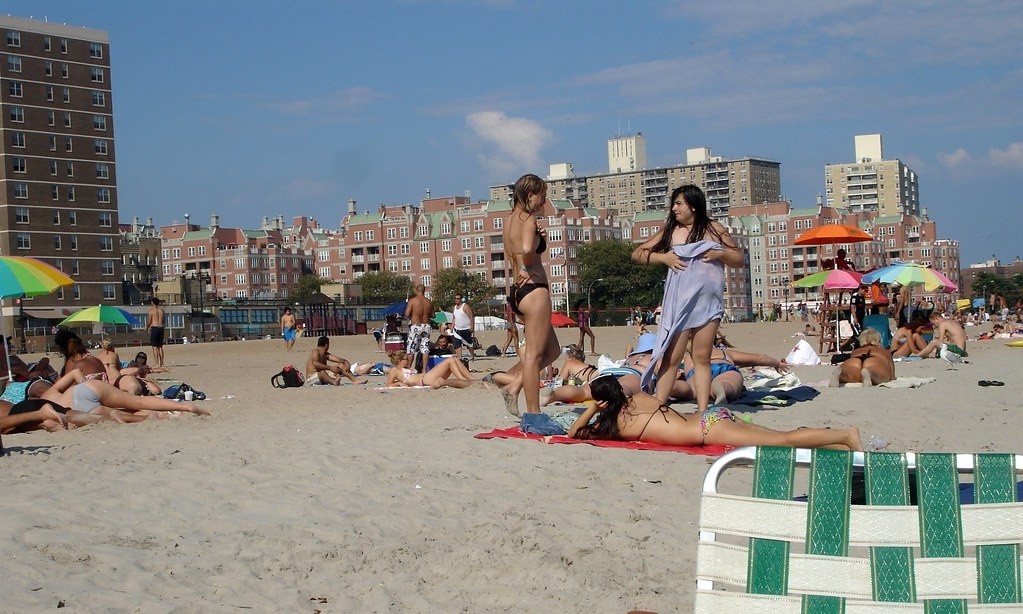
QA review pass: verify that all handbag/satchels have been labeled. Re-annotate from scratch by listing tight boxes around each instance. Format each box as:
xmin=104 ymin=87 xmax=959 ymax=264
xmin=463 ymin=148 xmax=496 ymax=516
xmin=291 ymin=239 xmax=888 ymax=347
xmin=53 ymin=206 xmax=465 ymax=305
xmin=164 ymin=383 xmax=206 ymax=400
xmin=786 ymin=339 xmax=821 ymax=365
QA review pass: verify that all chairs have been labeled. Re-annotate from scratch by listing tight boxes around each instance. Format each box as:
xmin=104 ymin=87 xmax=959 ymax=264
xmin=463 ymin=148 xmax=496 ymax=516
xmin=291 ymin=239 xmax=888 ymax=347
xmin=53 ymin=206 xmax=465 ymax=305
xmin=694 ymin=448 xmax=1023 ymax=614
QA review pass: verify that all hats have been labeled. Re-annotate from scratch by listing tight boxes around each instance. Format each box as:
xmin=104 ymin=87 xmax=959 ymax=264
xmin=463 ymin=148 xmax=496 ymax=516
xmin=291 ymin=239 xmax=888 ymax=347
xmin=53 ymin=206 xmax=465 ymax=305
xmin=628 ymin=334 xmax=657 ymax=355
xmin=987 ymin=331 xmax=994 ymax=337
xmin=0 ymin=334 xmax=12 ymax=345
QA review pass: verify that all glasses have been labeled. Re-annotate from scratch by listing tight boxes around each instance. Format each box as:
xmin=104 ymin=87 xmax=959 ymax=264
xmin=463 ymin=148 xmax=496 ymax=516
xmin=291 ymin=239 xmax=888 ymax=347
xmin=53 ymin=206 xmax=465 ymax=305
xmin=401 ymin=357 xmax=409 ymax=360
xmin=455 ymin=297 xmax=460 ymax=300
xmin=995 ymin=328 xmax=998 ymax=332
xmin=138 ymin=355 xmax=145 ymax=359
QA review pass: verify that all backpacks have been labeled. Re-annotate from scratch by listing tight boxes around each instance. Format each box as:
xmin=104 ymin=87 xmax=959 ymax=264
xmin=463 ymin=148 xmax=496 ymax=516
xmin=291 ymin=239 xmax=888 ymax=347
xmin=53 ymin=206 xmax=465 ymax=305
xmin=271 ymin=365 xmax=306 ymax=388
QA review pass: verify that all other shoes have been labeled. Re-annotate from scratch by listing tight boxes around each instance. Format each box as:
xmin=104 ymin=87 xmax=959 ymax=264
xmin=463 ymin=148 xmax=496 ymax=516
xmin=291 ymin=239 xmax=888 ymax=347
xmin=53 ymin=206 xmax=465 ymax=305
xmin=978 ymin=380 xmax=1005 ymax=386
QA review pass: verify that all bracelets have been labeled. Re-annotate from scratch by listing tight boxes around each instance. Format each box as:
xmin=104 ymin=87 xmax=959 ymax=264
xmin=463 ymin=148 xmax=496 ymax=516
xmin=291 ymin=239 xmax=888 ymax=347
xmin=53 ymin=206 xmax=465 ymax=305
xmin=521 ymin=266 xmax=531 ymax=271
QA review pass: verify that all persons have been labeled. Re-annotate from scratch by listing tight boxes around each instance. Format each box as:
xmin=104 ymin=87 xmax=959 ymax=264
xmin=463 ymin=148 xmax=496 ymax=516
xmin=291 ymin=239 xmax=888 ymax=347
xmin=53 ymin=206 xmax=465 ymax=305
xmin=281 ymin=306 xmax=296 ymax=350
xmin=568 ymin=373 xmax=864 ymax=452
xmin=371 ymin=284 xmax=483 ymax=389
xmin=827 ymin=329 xmax=896 ymax=387
xmin=501 ymin=174 xmax=560 ymax=417
xmin=334 ymin=361 xmax=394 ymax=375
xmin=770 ymin=249 xmax=1023 ymax=362
xmin=144 ymin=298 xmax=165 ymax=368
xmin=305 ymin=337 xmax=368 ymax=386
xmin=0 ymin=330 xmax=211 ymax=431
xmin=631 ymin=186 xmax=743 ymax=413
xmin=485 ymin=299 xmax=792 ymax=405
xmin=210 ymin=333 xmax=271 ymax=342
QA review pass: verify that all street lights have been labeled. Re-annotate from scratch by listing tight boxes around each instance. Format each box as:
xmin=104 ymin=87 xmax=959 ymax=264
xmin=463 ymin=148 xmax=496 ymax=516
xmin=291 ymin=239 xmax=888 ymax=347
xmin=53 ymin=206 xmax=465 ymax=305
xmin=191 ymin=268 xmax=210 ymax=344
xmin=780 ymin=277 xmax=789 ymax=322
xmin=405 ymin=277 xmax=424 ymax=303
xmin=587 ymin=279 xmax=605 ymax=326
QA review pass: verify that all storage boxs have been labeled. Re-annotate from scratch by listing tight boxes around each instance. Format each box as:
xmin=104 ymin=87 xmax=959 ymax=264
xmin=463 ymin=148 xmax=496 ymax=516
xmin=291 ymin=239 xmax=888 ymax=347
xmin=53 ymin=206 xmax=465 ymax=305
xmin=426 ymin=354 xmax=458 ymax=373
xmin=384 ymin=340 xmax=405 ymax=353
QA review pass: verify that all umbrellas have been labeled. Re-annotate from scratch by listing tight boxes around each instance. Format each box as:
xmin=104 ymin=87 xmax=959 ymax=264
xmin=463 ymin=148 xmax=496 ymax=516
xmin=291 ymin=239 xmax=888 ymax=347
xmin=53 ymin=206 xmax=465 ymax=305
xmin=380 ymin=299 xmax=408 ymax=330
xmin=0 ymin=254 xmax=74 ymax=380
xmin=518 ymin=312 xmax=577 ymax=326
xmin=58 ymin=305 xmax=141 ymax=341
xmin=793 ymin=224 xmax=958 ymax=354
xmin=430 ymin=311 xmax=453 ymax=324
